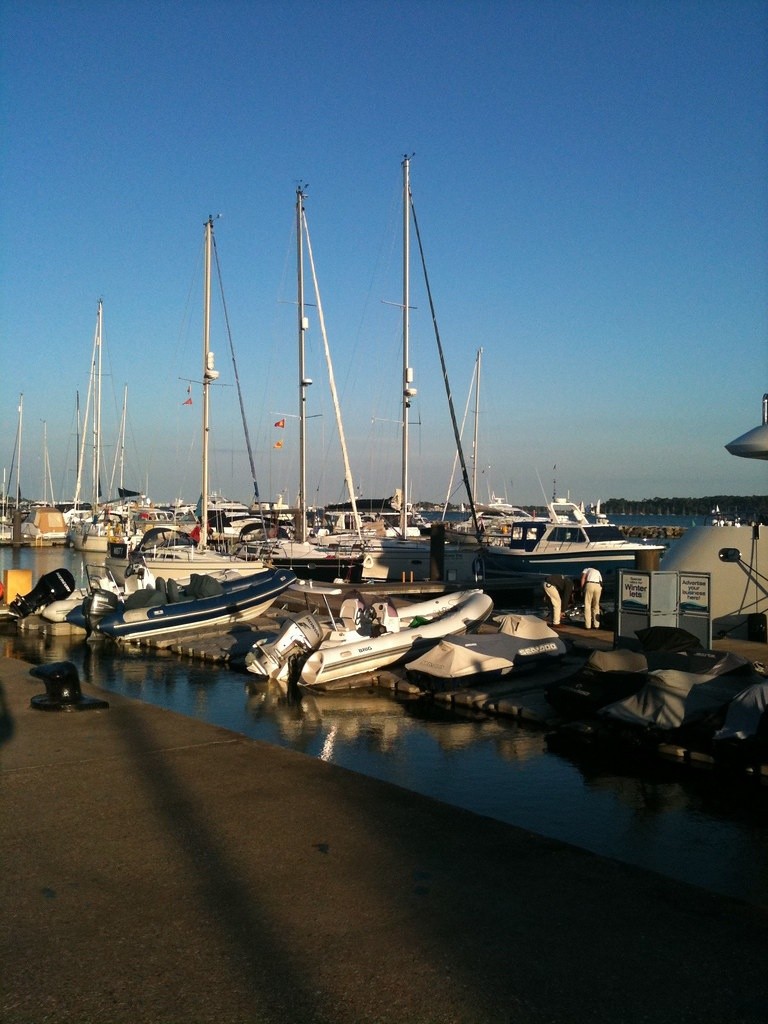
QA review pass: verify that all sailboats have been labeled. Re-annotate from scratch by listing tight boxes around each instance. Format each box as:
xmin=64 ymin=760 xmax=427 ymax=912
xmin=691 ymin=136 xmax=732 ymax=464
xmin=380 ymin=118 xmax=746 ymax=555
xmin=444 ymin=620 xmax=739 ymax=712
xmin=0 ymin=148 xmax=768 ymax=774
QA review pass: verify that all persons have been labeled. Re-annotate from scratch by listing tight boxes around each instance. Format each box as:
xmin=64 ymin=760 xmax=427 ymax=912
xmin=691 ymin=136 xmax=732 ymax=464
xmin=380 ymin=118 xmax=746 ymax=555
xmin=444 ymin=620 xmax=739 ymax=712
xmin=190 ymin=521 xmax=200 ymax=545
xmin=580 ymin=567 xmax=603 ymax=630
xmin=542 ymin=574 xmax=574 ymax=629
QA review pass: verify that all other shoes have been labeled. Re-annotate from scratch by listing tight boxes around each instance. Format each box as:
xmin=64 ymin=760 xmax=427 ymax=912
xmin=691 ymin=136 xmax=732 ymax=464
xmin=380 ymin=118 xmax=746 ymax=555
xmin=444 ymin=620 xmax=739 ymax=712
xmin=553 ymin=624 xmax=566 ymax=628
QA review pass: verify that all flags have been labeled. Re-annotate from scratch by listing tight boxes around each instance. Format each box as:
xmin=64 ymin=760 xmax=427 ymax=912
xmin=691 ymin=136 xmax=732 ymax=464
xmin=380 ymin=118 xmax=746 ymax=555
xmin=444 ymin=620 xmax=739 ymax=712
xmin=272 ymin=439 xmax=283 ymax=448
xmin=274 ymin=419 xmax=285 ymax=428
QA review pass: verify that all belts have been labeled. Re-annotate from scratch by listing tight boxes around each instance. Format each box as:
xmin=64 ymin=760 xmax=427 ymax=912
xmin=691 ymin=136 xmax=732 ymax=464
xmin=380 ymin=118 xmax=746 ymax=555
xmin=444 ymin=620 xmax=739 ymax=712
xmin=586 ymin=581 xmax=601 ymax=583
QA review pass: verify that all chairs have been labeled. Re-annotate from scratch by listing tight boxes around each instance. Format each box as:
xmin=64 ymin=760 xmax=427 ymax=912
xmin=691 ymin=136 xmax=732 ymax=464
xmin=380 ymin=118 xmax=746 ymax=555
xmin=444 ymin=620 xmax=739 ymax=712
xmin=372 ymin=593 xmax=411 ymax=633
xmin=340 ymin=589 xmax=365 ymax=622
xmin=155 ymin=577 xmax=168 ymax=600
xmin=166 ymin=578 xmax=196 ymax=603
xmin=90 ymin=573 xmax=122 ymax=595
